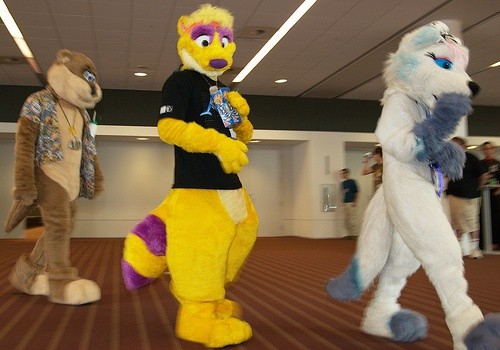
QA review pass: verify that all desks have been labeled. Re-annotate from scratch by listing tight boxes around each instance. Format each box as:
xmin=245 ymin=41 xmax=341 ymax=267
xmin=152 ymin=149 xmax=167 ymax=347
xmin=477 ymin=182 xmax=500 ymax=254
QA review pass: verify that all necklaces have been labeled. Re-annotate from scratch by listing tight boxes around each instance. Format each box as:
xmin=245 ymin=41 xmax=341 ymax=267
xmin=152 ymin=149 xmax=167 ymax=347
xmin=55 ymin=96 xmax=82 ymax=150
xmin=201 ymin=73 xmax=219 ymax=94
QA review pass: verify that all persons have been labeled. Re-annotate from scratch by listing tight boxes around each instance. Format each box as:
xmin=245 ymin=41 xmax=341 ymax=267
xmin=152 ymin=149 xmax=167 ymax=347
xmin=339 ymin=168 xmax=360 ymax=240
xmin=441 ymin=136 xmax=500 ymax=260
xmin=362 ymin=147 xmax=383 ymax=192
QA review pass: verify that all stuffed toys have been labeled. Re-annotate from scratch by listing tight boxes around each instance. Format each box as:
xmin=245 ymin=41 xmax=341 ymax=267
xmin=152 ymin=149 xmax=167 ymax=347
xmin=3 ymin=48 xmax=103 ymax=305
xmin=121 ymin=3 xmax=259 ymax=347
xmin=325 ymin=20 xmax=500 ymax=350
